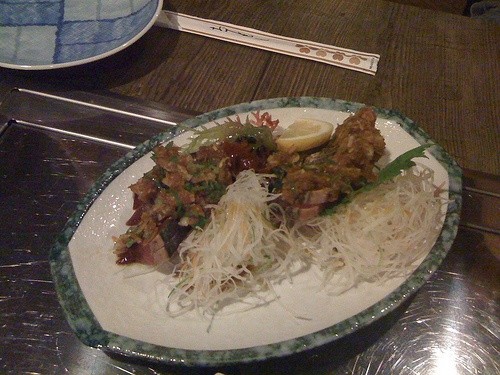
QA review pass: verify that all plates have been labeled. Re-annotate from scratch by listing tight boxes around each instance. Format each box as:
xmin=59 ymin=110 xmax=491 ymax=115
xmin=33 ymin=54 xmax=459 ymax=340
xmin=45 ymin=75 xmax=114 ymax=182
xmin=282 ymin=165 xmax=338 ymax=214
xmin=46 ymin=98 xmax=463 ymax=367
xmin=0 ymin=0 xmax=164 ymax=70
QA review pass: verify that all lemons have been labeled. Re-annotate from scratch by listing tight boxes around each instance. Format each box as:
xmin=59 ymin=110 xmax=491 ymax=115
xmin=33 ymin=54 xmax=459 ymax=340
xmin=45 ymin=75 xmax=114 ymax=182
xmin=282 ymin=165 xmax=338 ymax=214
xmin=276 ymin=119 xmax=334 ymax=151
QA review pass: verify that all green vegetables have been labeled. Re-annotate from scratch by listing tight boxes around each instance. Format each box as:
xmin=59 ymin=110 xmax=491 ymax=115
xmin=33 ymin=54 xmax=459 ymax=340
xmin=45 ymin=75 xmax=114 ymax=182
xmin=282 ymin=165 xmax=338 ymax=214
xmin=144 ymin=111 xmax=434 ymax=237
